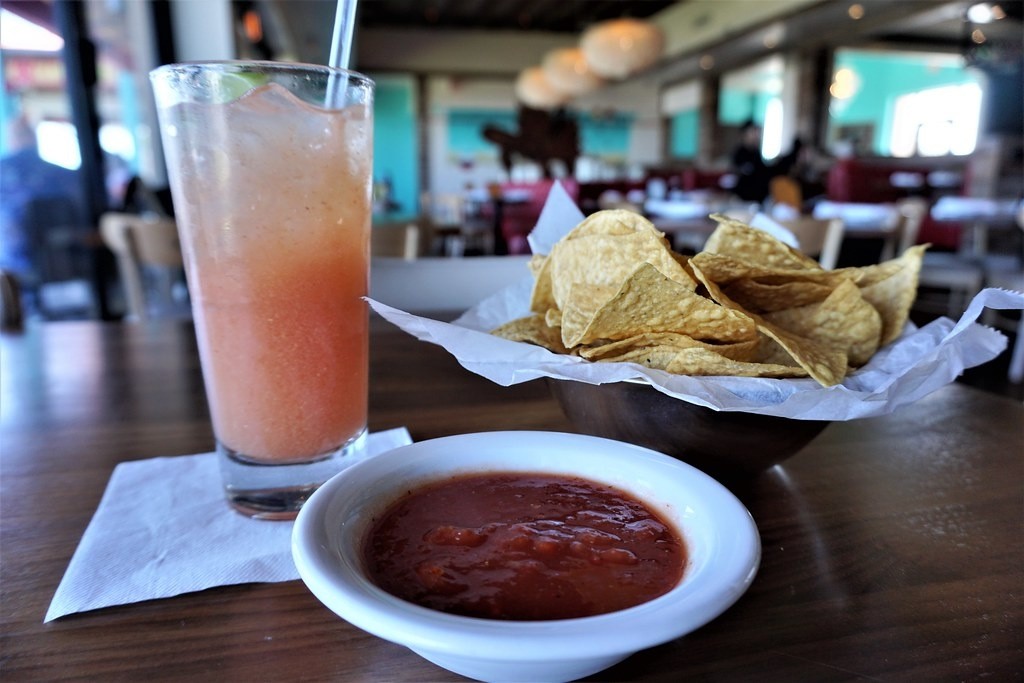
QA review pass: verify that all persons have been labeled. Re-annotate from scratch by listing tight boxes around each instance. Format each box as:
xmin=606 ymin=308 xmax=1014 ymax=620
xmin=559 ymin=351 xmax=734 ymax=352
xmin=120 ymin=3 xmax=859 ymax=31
xmin=70 ymin=114 xmax=170 ymax=228
xmin=1 ymin=116 xmax=129 ymax=324
xmin=730 ymin=117 xmax=804 ymax=204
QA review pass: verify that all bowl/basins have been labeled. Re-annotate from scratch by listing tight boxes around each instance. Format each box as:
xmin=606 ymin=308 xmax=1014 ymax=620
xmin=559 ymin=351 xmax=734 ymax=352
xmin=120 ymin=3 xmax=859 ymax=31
xmin=545 ymin=359 xmax=837 ymax=482
xmin=288 ymin=430 xmax=762 ymax=682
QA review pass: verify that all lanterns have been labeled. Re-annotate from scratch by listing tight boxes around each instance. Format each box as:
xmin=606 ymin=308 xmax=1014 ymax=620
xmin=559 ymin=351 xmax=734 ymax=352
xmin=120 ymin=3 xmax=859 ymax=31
xmin=543 ymin=44 xmax=614 ymax=96
xmin=578 ymin=18 xmax=666 ymax=84
xmin=515 ymin=66 xmax=574 ymax=113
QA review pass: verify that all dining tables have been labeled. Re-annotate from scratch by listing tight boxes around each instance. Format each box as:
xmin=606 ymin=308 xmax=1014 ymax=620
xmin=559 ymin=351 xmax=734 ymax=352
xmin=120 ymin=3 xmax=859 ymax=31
xmin=0 ymin=286 xmax=1024 ymax=683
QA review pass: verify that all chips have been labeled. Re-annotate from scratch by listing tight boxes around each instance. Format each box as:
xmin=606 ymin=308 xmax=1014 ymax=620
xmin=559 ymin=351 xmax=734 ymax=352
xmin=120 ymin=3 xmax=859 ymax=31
xmin=490 ymin=209 xmax=930 ymax=392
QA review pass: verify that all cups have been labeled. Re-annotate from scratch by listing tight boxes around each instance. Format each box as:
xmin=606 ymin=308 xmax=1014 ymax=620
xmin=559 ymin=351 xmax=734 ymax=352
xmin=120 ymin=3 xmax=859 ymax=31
xmin=146 ymin=62 xmax=375 ymax=522
